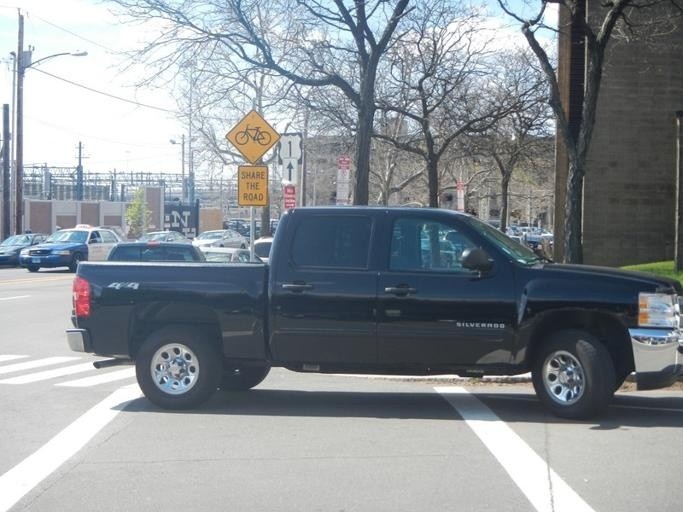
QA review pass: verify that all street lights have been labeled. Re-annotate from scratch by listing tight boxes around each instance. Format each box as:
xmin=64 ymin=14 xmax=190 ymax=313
xmin=168 ymin=138 xmax=187 ymax=203
xmin=179 ymin=60 xmax=195 ymax=199
xmin=14 ymin=49 xmax=89 ymax=235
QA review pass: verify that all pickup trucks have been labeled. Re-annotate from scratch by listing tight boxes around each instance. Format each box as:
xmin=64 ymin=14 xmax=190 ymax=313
xmin=60 ymin=200 xmax=682 ymax=420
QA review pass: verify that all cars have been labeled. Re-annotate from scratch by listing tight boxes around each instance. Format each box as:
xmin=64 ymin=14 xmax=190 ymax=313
xmin=95 ymin=217 xmax=280 ymax=265
xmin=407 ymin=209 xmax=556 ymax=270
xmin=0 ymin=231 xmax=49 ymax=267
xmin=17 ymin=222 xmax=122 ymax=274
